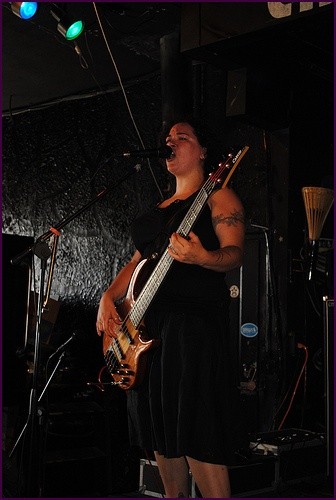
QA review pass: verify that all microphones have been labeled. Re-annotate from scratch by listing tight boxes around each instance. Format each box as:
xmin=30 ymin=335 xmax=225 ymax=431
xmin=48 ymin=328 xmax=81 ymax=359
xmin=123 ymin=145 xmax=172 ymax=159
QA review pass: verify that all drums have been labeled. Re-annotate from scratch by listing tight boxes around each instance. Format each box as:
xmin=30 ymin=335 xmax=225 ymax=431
xmin=302 ymin=187 xmax=334 ymax=243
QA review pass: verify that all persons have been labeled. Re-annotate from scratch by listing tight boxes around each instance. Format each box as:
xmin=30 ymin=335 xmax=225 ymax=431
xmin=94 ymin=115 xmax=247 ymax=499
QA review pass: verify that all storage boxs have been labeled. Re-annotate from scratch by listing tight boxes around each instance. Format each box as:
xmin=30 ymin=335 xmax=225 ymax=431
xmin=138 ymin=453 xmax=279 ymax=500
xmin=249 ymin=427 xmax=324 ymax=485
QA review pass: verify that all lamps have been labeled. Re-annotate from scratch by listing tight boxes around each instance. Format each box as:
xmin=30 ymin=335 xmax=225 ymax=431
xmin=49 ymin=7 xmax=85 ymax=40
xmin=2 ymin=1 xmax=37 ymax=20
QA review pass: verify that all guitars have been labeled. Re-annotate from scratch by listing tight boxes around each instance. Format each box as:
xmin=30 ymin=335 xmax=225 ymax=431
xmin=102 ymin=145 xmax=250 ymax=390
